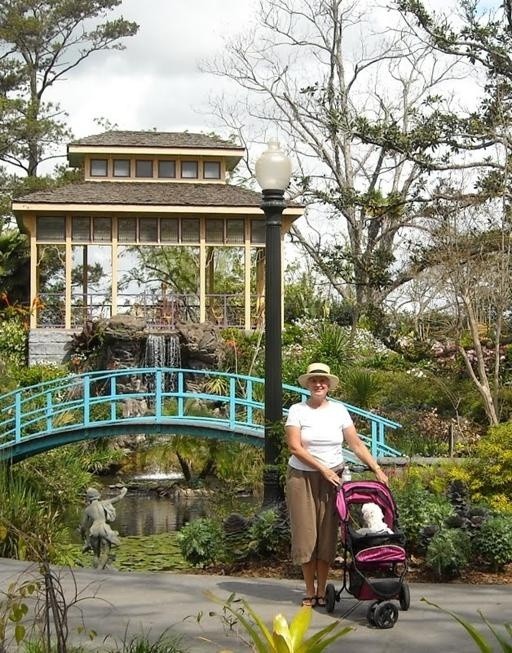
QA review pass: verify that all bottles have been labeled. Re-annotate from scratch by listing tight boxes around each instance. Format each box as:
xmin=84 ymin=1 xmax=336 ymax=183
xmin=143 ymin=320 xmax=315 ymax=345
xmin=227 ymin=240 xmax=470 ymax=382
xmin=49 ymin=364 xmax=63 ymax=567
xmin=342 ymin=466 xmax=352 ymax=483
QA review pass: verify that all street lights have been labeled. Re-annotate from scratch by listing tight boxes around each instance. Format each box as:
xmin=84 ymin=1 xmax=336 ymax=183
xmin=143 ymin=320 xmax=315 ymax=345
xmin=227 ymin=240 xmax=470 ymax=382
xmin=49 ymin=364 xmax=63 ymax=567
xmin=253 ymin=132 xmax=296 ymax=524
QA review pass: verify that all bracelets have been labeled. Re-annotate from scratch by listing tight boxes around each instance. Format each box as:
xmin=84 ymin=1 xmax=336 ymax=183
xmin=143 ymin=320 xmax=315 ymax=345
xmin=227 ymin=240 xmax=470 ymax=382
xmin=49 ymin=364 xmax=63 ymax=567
xmin=374 ymin=467 xmax=383 ymax=474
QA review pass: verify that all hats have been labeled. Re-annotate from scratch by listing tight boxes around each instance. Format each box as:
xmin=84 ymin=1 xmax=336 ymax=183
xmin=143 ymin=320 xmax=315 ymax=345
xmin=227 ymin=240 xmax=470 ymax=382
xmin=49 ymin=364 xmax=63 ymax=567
xmin=297 ymin=362 xmax=339 ymax=391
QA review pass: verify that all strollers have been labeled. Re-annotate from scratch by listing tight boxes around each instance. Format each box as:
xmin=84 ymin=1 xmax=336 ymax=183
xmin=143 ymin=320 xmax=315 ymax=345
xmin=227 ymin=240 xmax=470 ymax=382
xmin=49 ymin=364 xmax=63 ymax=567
xmin=322 ymin=464 xmax=417 ymax=627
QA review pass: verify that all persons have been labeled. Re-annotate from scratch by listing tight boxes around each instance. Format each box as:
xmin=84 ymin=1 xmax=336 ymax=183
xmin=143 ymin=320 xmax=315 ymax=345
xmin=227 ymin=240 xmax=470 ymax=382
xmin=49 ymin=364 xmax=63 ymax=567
xmin=81 ymin=484 xmax=130 ymax=572
xmin=282 ymin=358 xmax=391 ymax=610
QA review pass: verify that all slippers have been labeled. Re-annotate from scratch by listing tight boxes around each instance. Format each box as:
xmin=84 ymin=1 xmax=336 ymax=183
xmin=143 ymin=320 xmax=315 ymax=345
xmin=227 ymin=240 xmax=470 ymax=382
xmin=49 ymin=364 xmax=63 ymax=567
xmin=317 ymin=592 xmax=329 ymax=607
xmin=302 ymin=595 xmax=317 ymax=608
